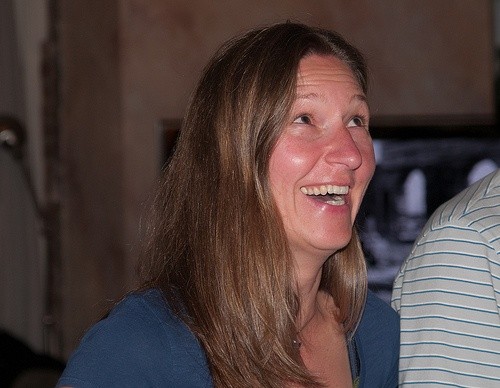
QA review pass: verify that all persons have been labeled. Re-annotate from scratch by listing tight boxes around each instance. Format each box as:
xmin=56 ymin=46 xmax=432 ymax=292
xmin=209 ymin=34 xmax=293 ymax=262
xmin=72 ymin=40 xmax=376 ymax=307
xmin=54 ymin=20 xmax=400 ymax=388
xmin=391 ymin=168 xmax=500 ymax=388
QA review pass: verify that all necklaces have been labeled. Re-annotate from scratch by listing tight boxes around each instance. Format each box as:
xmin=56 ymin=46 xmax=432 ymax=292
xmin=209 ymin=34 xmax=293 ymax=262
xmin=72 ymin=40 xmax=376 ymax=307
xmin=296 ymin=302 xmax=318 ymax=348
